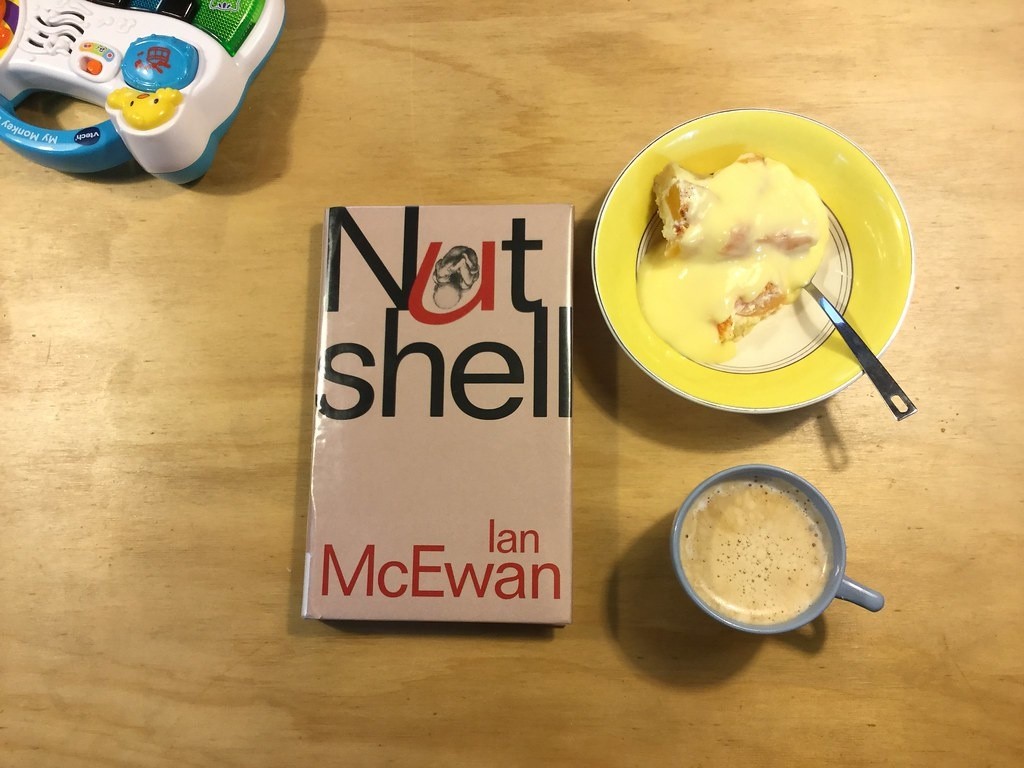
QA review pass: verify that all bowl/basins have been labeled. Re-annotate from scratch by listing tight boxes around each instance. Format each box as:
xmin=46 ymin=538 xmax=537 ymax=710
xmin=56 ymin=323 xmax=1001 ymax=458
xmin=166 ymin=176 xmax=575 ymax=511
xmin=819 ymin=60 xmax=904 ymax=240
xmin=591 ymin=108 xmax=917 ymax=416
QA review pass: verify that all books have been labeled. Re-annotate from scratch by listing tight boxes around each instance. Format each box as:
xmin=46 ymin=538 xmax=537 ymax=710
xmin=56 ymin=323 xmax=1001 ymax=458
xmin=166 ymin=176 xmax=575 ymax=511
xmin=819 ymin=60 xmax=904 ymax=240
xmin=300 ymin=205 xmax=573 ymax=625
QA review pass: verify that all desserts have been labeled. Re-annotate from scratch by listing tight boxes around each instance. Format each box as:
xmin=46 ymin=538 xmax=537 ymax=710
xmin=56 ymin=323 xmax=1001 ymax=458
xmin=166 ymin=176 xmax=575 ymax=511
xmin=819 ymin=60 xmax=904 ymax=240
xmin=653 ymin=152 xmax=828 ymax=345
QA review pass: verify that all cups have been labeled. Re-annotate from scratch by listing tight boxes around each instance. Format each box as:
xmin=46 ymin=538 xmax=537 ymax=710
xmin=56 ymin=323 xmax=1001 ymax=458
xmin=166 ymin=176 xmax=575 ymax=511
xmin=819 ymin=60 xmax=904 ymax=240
xmin=670 ymin=464 xmax=884 ymax=635
